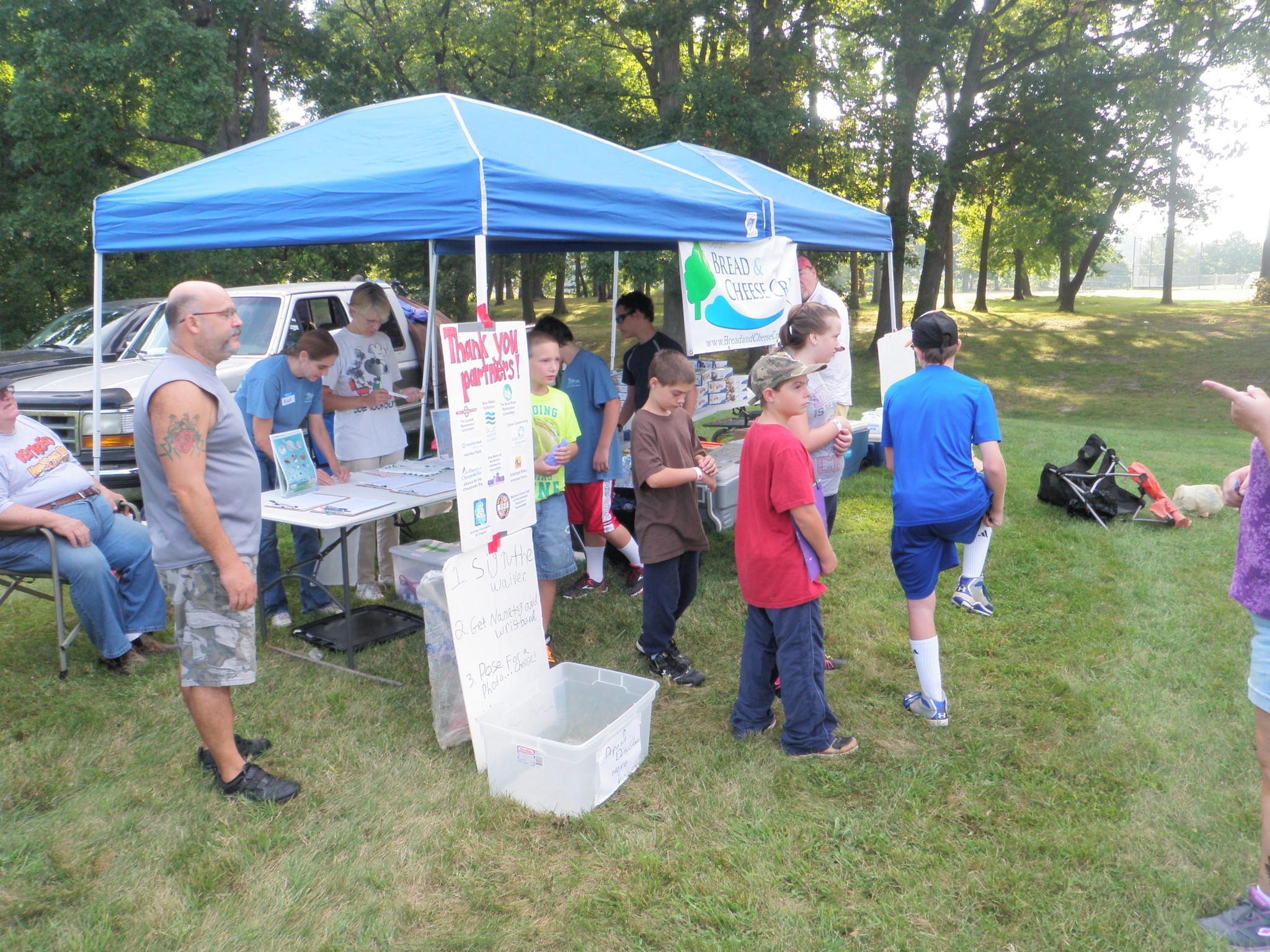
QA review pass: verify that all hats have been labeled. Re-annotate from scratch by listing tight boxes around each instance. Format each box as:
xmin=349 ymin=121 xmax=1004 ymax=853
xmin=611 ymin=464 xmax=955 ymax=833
xmin=905 ymin=309 xmax=958 ymax=349
xmin=796 ymin=255 xmax=812 ymax=269
xmin=0 ymin=375 xmax=18 ymax=389
xmin=747 ymin=351 xmax=827 ymax=406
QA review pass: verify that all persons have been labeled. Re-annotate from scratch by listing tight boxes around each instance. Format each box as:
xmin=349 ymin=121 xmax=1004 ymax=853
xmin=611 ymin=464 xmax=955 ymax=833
xmin=132 ymin=280 xmax=300 ymax=805
xmin=320 ymin=281 xmax=428 ymax=601
xmin=630 ymin=349 xmax=718 ymax=688
xmin=786 ymin=255 xmax=850 ymax=429
xmin=762 ymin=302 xmax=851 ymax=702
xmin=0 ymin=374 xmax=180 ymax=679
xmin=1198 ymin=361 xmax=1269 ymax=948
xmin=230 ymin=329 xmax=352 ymax=627
xmin=611 ymin=291 xmax=697 ymax=437
xmin=526 ymin=330 xmax=582 ymax=667
xmin=882 ymin=311 xmax=1007 ymax=731
xmin=531 ymin=315 xmax=648 ymax=600
xmin=731 ymin=351 xmax=860 ymax=760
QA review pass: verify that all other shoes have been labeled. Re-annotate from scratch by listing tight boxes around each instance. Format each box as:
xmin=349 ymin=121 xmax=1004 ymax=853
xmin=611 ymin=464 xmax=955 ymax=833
xmin=212 ymin=762 xmax=301 ymax=804
xmin=318 ymin=605 xmax=343 ymax=614
xmin=196 ymin=734 xmax=272 ymax=770
xmin=270 ymin=612 xmax=292 ymax=628
xmin=378 ymin=573 xmax=395 ymax=586
xmin=99 ymin=646 xmax=148 ymax=675
xmin=131 ymin=634 xmax=177 ymax=654
xmin=356 ymin=581 xmax=386 ymax=602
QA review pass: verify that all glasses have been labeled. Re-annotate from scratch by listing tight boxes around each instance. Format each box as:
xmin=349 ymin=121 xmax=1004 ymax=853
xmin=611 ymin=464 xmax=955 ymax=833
xmin=0 ymin=385 xmax=15 ymax=399
xmin=179 ymin=308 xmax=237 ymax=324
xmin=615 ymin=308 xmax=643 ymax=324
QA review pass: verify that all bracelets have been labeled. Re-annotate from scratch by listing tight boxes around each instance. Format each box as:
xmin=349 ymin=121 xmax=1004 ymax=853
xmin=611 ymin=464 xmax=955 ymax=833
xmin=829 ymin=417 xmax=846 ymax=434
xmin=97 ymin=483 xmax=106 ymax=491
xmin=694 ymin=465 xmax=704 ymax=485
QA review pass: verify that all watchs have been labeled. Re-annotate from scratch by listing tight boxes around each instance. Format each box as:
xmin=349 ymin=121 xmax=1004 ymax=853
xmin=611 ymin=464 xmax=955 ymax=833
xmin=616 ymin=421 xmax=624 ymax=433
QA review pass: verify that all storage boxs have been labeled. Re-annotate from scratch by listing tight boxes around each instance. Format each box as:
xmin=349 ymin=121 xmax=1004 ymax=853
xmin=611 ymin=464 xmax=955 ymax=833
xmin=473 ymin=661 xmax=662 ymax=818
xmin=842 ymin=419 xmax=873 ymax=478
xmin=389 ymin=537 xmax=459 ymax=606
xmin=609 ymin=357 xmax=747 ymax=411
xmin=697 ymin=442 xmax=752 ymax=533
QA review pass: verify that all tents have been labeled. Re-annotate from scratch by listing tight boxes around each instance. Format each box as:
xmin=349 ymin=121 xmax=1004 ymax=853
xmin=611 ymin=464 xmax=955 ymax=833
xmin=90 ymin=90 xmax=776 ymax=487
xmin=424 ymin=136 xmax=897 ymax=410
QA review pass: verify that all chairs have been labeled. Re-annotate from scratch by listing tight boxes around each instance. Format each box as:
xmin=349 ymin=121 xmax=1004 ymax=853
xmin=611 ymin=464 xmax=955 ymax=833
xmin=0 ymin=500 xmax=144 ymax=683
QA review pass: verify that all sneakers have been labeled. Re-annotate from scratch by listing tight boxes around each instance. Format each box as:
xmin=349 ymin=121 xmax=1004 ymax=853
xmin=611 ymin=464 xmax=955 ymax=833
xmin=903 ymin=690 xmax=949 ymax=728
xmin=649 ymin=651 xmax=706 ymax=686
xmin=763 ymin=716 xmax=776 ymax=731
xmin=620 ymin=563 xmax=644 ymax=597
xmin=787 ymin=736 xmax=858 ymax=758
xmin=773 ymin=678 xmax=781 ymax=700
xmin=825 ymin=653 xmax=845 ymax=672
xmin=561 ymin=572 xmax=608 ymax=600
xmin=951 ymin=572 xmax=994 ymax=617
xmin=635 ymin=631 xmax=692 ymax=666
xmin=1195 ymin=884 xmax=1270 ymax=951
xmin=544 ymin=633 xmax=555 ymax=669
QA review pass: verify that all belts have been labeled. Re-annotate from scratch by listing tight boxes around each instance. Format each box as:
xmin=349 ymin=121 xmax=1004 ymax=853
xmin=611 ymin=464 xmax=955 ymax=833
xmin=35 ymin=487 xmax=103 ymax=510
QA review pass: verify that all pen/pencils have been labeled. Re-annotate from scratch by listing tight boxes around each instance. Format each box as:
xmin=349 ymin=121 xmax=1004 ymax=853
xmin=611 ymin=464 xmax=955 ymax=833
xmin=364 ymin=482 xmax=386 ymax=487
xmin=318 ymin=505 xmax=349 ymax=513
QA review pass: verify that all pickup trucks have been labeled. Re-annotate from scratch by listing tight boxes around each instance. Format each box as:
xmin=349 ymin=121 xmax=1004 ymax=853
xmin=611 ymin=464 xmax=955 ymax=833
xmin=0 ymin=281 xmax=449 ymax=510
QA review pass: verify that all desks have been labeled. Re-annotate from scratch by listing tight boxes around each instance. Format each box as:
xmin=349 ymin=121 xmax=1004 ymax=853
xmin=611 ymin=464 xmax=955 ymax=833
xmin=258 ymin=458 xmax=458 ymax=671
xmin=711 ymin=395 xmax=764 ymax=412
xmin=620 ymin=406 xmax=718 ymax=453
